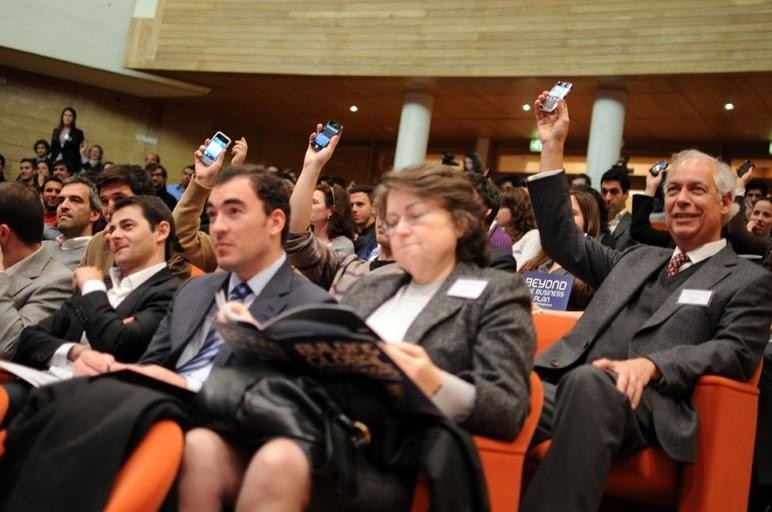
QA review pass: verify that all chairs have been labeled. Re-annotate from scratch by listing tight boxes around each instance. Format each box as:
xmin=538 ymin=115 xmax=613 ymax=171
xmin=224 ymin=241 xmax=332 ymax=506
xmin=1 ymin=378 xmax=183 ymax=512
xmin=411 ymin=313 xmax=761 ymax=512
xmin=191 ymin=265 xmax=205 ymax=276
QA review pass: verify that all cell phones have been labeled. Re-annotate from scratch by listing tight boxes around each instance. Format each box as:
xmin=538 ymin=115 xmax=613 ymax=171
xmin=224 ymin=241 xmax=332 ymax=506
xmin=650 ymin=160 xmax=668 ymax=176
xmin=539 ymin=81 xmax=572 ymax=113
xmin=200 ymin=131 xmax=232 ymax=166
xmin=737 ymin=160 xmax=755 ymax=177
xmin=310 ymin=119 xmax=341 ymax=150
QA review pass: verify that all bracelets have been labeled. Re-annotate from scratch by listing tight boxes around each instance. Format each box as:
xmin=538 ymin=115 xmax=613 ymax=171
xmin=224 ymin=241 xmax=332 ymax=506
xmin=424 ymin=376 xmax=446 ymax=401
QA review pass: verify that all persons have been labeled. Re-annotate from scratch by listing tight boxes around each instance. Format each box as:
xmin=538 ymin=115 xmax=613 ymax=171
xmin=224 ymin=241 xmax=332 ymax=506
xmin=524 ymin=80 xmax=771 ymax=510
xmin=70 ymin=163 xmax=338 ymax=392
xmin=172 ymin=162 xmax=538 ymax=512
xmin=50 ymin=106 xmax=85 ymax=174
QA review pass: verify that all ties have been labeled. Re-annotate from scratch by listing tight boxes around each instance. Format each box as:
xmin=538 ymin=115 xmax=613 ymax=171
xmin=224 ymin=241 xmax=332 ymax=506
xmin=170 ymin=280 xmax=255 ymax=378
xmin=665 ymin=251 xmax=692 ymax=279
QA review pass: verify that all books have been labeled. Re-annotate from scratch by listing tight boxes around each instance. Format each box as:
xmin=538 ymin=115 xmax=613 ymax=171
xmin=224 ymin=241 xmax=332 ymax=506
xmin=210 ymin=286 xmax=447 ymax=425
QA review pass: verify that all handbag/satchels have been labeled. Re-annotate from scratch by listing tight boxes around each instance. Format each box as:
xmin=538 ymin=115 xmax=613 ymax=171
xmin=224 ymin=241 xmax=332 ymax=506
xmin=185 ymin=346 xmax=386 ymax=454
